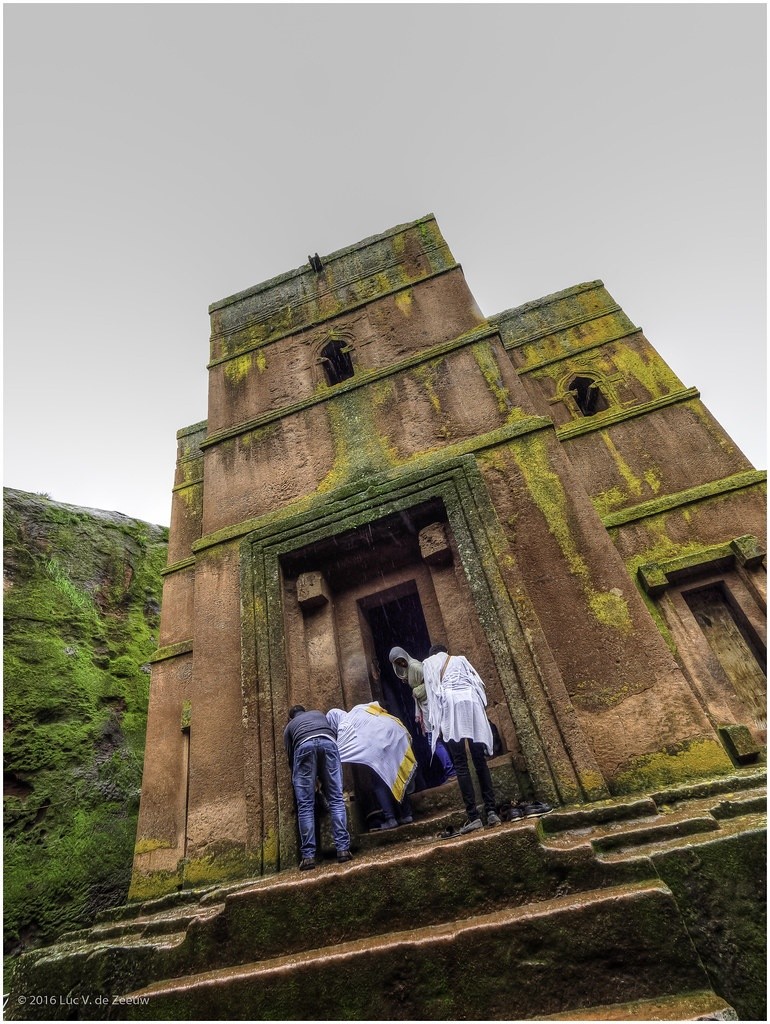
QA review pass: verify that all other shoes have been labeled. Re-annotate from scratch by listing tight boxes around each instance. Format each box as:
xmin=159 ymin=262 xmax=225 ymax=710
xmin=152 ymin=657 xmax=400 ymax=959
xmin=440 ymin=776 xmax=457 ymax=786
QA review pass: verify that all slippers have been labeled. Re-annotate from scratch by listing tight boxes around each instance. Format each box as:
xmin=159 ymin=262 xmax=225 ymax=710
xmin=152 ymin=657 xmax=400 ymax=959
xmin=439 ymin=825 xmax=459 ymax=839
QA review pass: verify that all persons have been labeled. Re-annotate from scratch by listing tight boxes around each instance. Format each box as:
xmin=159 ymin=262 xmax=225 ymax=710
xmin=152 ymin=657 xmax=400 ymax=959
xmin=325 ymin=701 xmax=416 ymax=832
xmin=284 ymin=704 xmax=352 ymax=871
xmin=423 ymin=643 xmax=500 ymax=833
xmin=388 ymin=646 xmax=458 ymax=786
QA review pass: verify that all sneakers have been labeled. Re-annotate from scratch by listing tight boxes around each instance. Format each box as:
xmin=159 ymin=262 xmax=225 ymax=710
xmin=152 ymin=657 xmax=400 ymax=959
xmin=524 ymin=800 xmax=553 ymax=819
xmin=337 ymin=850 xmax=353 ymax=863
xmin=368 ymin=816 xmax=413 ymax=832
xmin=501 ymin=804 xmax=523 ymax=822
xmin=299 ymin=859 xmax=316 ymax=870
xmin=460 ymin=814 xmax=501 ymax=835
